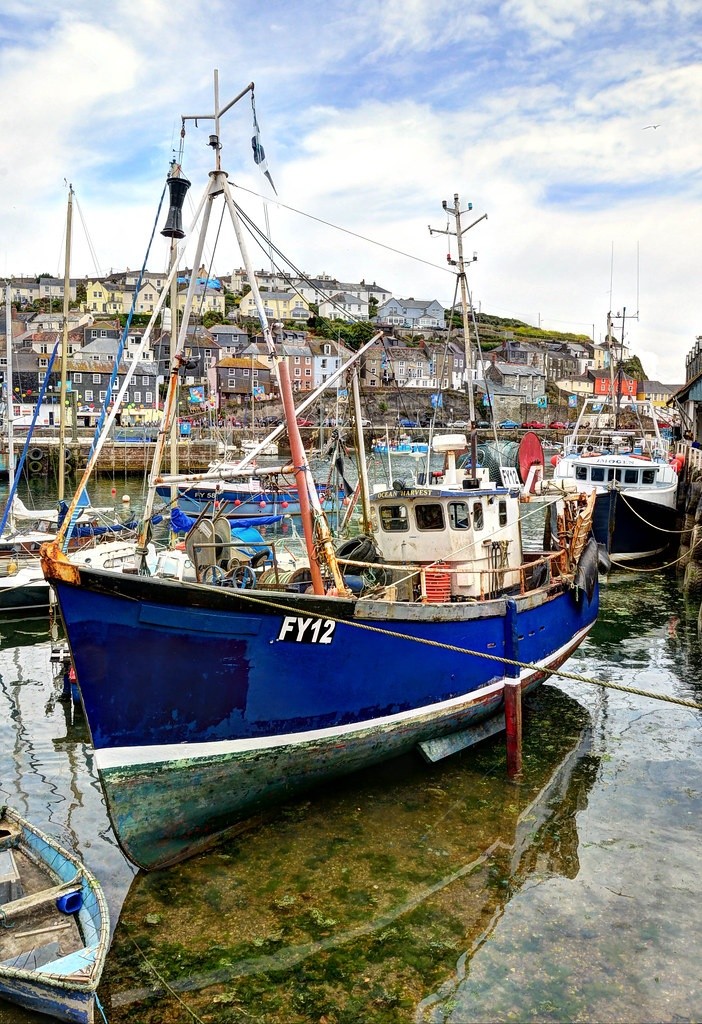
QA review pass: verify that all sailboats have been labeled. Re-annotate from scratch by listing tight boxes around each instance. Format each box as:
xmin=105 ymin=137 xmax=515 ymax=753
xmin=196 ymin=386 xmax=237 ymax=456
xmin=237 ymin=353 xmax=278 ymax=454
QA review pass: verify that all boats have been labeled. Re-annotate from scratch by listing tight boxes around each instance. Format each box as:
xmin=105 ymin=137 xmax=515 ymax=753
xmin=39 ymin=65 xmax=606 ymax=863
xmin=0 ymin=504 xmax=137 ymax=610
xmin=0 ymin=807 xmax=110 ymax=1024
xmin=371 ymin=431 xmax=428 ymax=455
xmin=549 ymin=241 xmax=682 ymax=569
xmin=0 ymin=449 xmax=18 ymax=472
xmin=153 ymin=463 xmax=349 ymax=517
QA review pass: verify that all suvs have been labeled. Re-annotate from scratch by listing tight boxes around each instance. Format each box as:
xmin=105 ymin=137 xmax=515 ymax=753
xmin=349 ymin=416 xmax=371 ymax=427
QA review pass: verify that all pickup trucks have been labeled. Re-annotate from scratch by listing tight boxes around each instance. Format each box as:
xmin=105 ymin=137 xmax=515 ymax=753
xmin=420 ymin=416 xmax=444 ymax=428
xmin=522 ymin=420 xmax=546 ymax=429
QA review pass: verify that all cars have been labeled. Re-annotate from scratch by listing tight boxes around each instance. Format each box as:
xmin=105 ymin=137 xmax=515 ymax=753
xmin=319 ymin=416 xmax=343 ymax=427
xmin=446 ymin=419 xmax=467 ymax=428
xmin=495 ymin=419 xmax=518 ymax=429
xmin=550 ymin=420 xmax=578 ymax=428
xmin=473 ymin=420 xmax=493 ymax=428
xmin=296 ymin=418 xmax=314 ymax=427
xmin=259 ymin=415 xmax=282 ymax=426
xmin=396 ymin=418 xmax=417 ymax=427
